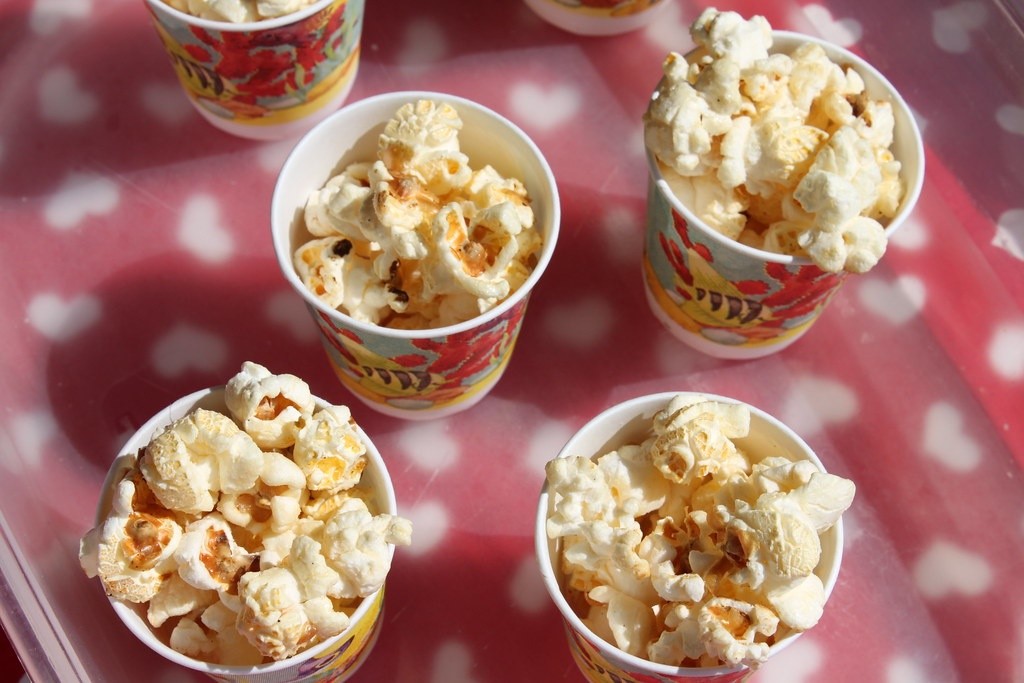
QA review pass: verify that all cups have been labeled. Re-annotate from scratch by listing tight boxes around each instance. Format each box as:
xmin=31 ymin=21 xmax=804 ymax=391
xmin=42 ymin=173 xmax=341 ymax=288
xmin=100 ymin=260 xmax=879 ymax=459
xmin=534 ymin=392 xmax=846 ymax=683
xmin=642 ymin=31 xmax=926 ymax=359
xmin=527 ymin=0 xmax=670 ymax=35
xmin=96 ymin=383 xmax=400 ymax=683
xmin=270 ymin=91 xmax=560 ymax=421
xmin=146 ymin=0 xmax=364 ymax=141
